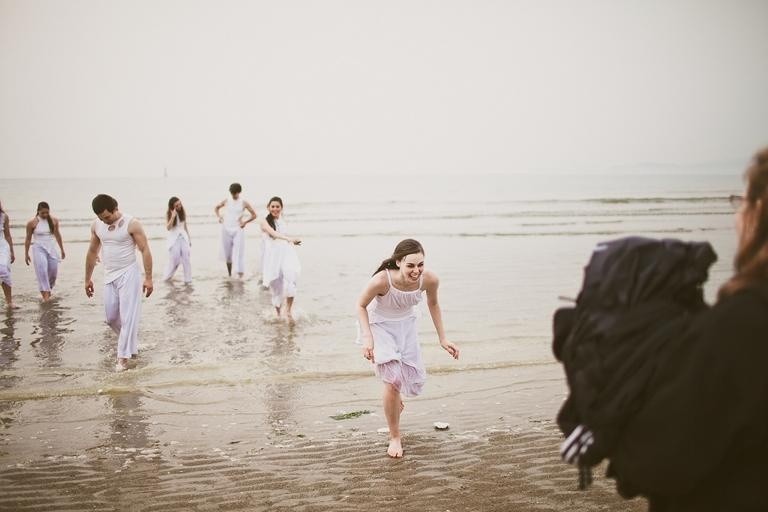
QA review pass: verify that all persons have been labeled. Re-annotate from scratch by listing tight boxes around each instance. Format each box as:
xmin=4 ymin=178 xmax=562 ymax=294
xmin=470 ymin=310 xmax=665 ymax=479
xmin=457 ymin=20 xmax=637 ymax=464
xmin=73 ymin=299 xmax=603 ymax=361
xmin=0 ymin=201 xmax=19 ymax=309
xmin=608 ymin=145 xmax=768 ymax=512
xmin=160 ymin=196 xmax=192 ymax=288
xmin=355 ymin=239 xmax=459 ymax=458
xmin=24 ymin=202 xmax=65 ymax=302
xmin=213 ymin=182 xmax=257 ymax=278
xmin=85 ymin=194 xmax=153 ymax=370
xmin=259 ymin=196 xmax=303 ymax=322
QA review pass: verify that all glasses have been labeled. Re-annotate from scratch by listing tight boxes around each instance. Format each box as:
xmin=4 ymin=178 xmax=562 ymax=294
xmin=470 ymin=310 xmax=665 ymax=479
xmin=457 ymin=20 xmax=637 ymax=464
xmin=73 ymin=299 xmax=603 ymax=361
xmin=727 ymin=191 xmax=758 ymax=209
